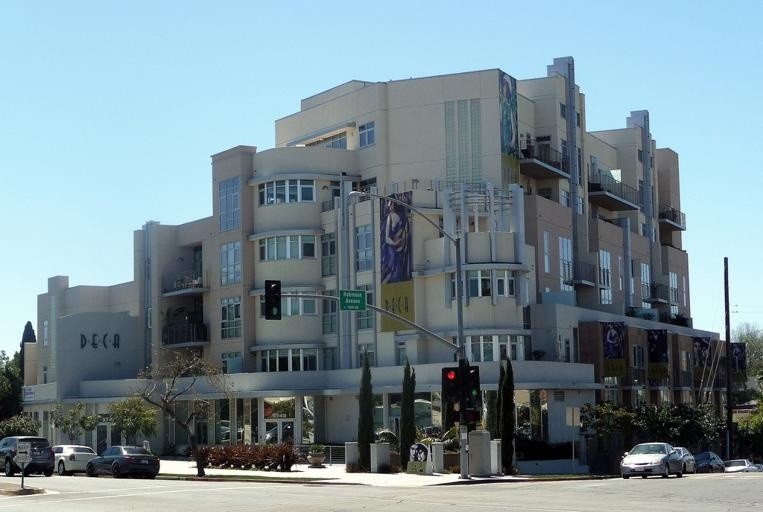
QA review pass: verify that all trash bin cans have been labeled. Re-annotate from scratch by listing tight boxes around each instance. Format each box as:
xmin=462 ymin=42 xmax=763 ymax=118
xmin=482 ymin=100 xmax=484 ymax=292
xmin=432 ymin=441 xmax=444 ymax=473
xmin=345 ymin=442 xmax=358 ymax=464
xmin=369 ymin=443 xmax=389 ymax=472
xmin=467 ymin=429 xmax=491 ymax=477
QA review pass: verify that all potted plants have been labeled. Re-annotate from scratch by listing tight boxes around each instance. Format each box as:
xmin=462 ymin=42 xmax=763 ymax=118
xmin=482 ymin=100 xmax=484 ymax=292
xmin=307 ymin=443 xmax=327 ymax=468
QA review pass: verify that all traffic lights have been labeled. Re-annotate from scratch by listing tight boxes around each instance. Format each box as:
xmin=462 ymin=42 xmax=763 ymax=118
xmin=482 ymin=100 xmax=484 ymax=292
xmin=442 ymin=366 xmax=481 ymax=422
xmin=264 ymin=279 xmax=281 ymax=319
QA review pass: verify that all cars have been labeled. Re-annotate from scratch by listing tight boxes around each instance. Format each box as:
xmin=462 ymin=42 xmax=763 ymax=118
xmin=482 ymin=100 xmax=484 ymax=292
xmin=724 ymin=458 xmax=758 ymax=472
xmin=51 ymin=444 xmax=97 ymax=475
xmin=619 ymin=441 xmax=682 ymax=478
xmin=84 ymin=445 xmax=159 ymax=478
xmin=693 ymin=451 xmax=724 ymax=472
xmin=673 ymin=446 xmax=697 ymax=473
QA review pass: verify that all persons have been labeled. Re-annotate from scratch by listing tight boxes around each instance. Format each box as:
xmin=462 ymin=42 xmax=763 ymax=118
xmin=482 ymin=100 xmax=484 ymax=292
xmin=693 ymin=338 xmax=710 ymax=368
xmin=605 ymin=322 xmax=620 ymax=359
xmin=382 ymin=202 xmax=409 ymax=284
xmin=502 ymin=75 xmax=516 ymax=157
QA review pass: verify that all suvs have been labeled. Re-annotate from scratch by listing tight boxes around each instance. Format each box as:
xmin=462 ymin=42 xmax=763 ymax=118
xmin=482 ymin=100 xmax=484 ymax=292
xmin=0 ymin=435 xmax=55 ymax=477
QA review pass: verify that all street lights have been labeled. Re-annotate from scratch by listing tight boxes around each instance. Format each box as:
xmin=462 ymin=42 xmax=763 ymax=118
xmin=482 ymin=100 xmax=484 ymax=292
xmin=349 ymin=187 xmax=472 ymax=479
